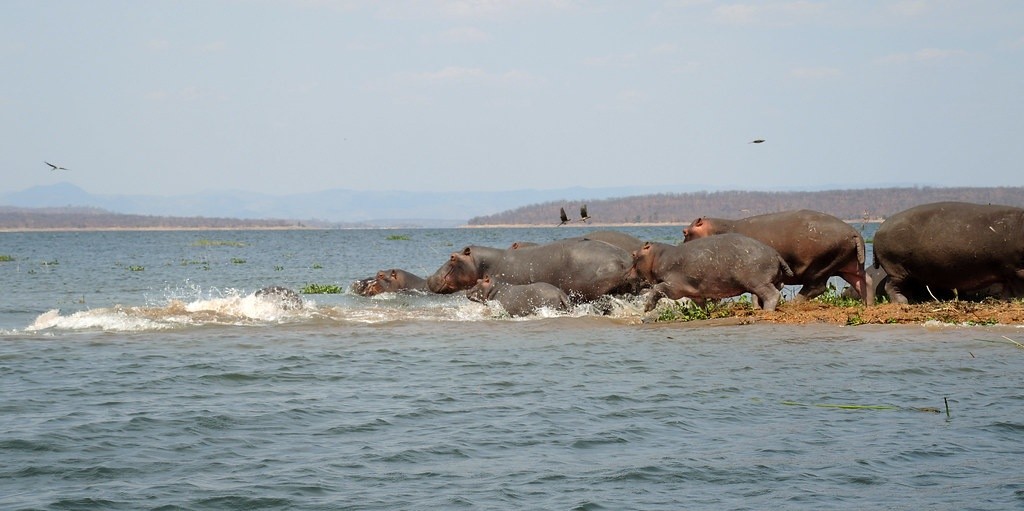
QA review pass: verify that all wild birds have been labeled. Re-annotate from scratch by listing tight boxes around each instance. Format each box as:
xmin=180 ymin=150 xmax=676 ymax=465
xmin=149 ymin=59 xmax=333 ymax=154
xmin=44 ymin=161 xmax=69 ymax=171
xmin=577 ymin=203 xmax=592 ymax=223
xmin=555 ymin=206 xmax=572 ymax=228
xmin=745 ymin=137 xmax=767 ymax=146
